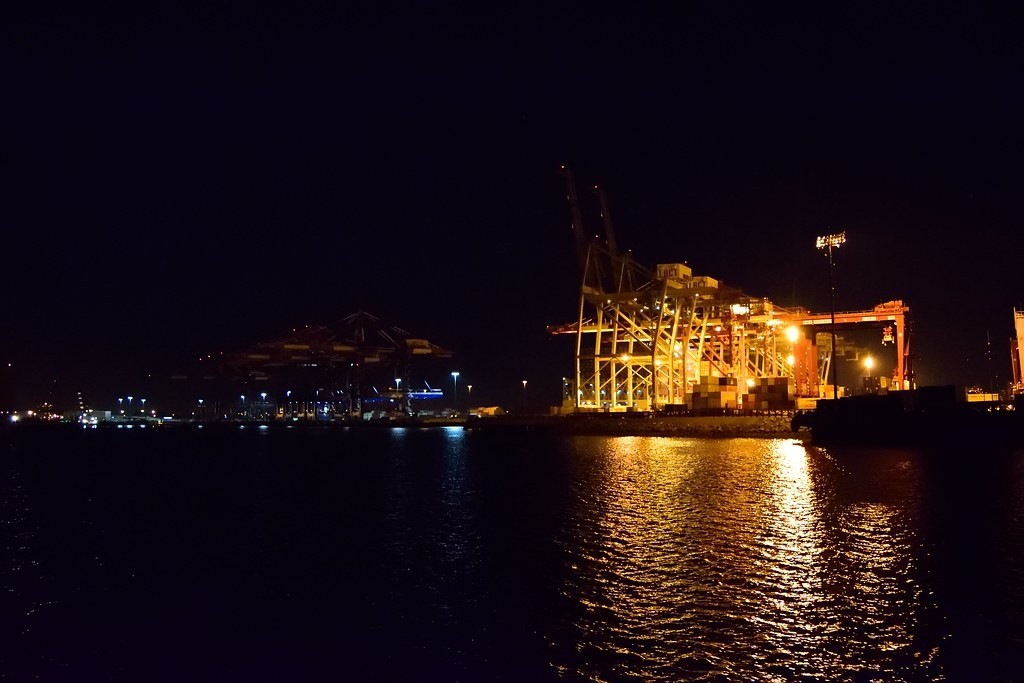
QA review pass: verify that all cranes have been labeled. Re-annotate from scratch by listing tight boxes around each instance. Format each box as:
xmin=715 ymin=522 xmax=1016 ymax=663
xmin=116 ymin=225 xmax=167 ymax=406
xmin=553 ymin=159 xmax=636 ymax=300
xmin=241 ymin=307 xmax=454 ymax=414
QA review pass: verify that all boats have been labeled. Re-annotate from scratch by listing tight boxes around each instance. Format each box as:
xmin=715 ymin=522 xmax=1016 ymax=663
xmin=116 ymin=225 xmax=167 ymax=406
xmin=788 ymin=382 xmax=1023 ymax=449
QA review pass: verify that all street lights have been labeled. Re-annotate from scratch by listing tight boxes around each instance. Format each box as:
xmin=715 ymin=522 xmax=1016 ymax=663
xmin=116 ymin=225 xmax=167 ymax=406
xmin=452 ymin=371 xmax=460 ymax=412
xmin=813 ymin=232 xmax=847 ymax=399
xmin=521 ymin=380 xmax=528 ymax=411
xmin=467 ymin=384 xmax=474 ymax=403
xmin=394 ymin=378 xmax=402 ymax=392
